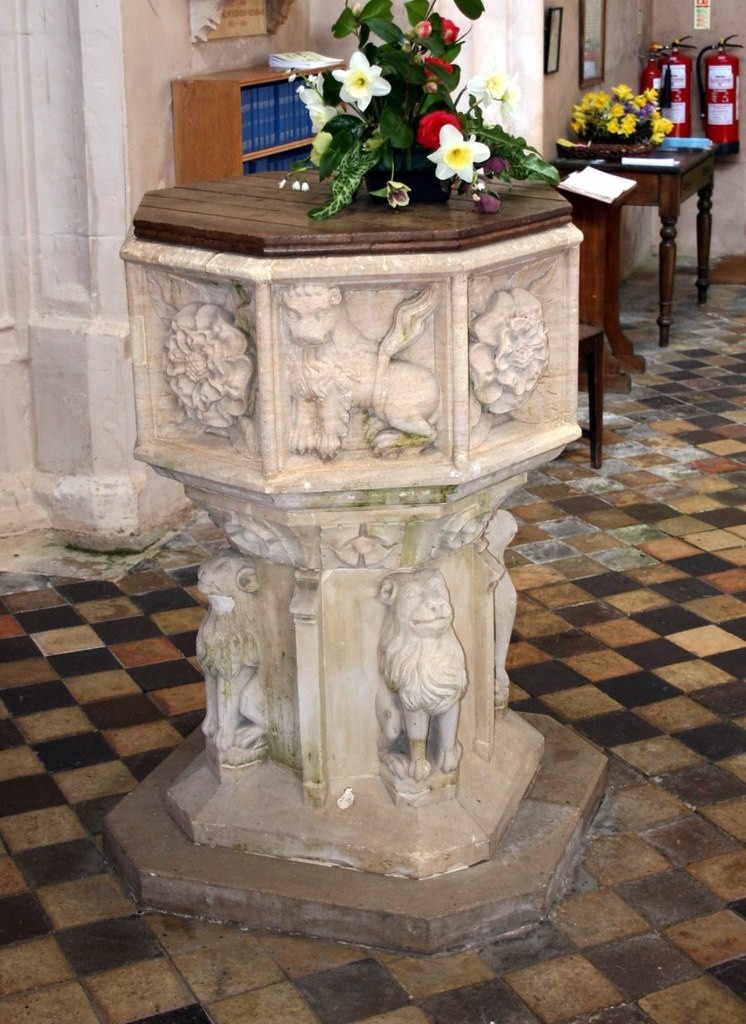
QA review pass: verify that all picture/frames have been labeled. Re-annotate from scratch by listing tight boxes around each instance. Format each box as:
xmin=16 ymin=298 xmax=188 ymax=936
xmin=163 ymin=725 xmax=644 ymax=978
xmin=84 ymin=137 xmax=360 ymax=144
xmin=544 ymin=6 xmax=563 ymax=75
xmin=578 ymin=0 xmax=607 ymax=90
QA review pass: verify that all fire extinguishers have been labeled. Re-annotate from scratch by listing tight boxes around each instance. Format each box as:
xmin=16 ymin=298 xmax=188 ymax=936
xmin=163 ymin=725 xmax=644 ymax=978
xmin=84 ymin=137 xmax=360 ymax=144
xmin=660 ymin=35 xmax=698 ymax=138
xmin=639 ymin=41 xmax=665 ymax=118
xmin=696 ymin=33 xmax=745 ymax=155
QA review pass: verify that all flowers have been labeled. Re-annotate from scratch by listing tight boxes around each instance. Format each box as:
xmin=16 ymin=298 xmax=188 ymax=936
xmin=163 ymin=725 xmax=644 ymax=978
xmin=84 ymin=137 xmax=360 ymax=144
xmin=571 ymin=81 xmax=675 ymax=143
xmin=278 ymin=0 xmax=564 ymax=227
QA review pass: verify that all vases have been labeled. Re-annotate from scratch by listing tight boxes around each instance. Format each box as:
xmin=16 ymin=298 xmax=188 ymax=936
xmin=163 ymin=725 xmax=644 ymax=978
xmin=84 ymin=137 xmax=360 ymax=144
xmin=365 ymin=124 xmax=461 ymax=204
xmin=568 ymin=127 xmax=654 ymax=144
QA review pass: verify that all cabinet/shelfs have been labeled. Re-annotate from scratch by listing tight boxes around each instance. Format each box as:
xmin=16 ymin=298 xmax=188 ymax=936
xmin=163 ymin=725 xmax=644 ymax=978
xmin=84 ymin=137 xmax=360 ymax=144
xmin=171 ymin=62 xmax=349 ymax=186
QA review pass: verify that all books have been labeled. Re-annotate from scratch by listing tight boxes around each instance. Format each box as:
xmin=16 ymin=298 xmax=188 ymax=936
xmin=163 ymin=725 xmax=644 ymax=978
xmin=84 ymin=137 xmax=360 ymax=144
xmin=268 ymin=50 xmax=345 ymax=71
xmin=555 ymin=165 xmax=637 ymax=206
xmin=241 ymin=81 xmax=319 ymax=176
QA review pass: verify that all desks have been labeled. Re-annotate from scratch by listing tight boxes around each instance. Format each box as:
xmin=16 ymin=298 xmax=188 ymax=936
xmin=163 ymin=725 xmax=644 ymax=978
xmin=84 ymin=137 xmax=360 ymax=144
xmin=543 ymin=143 xmax=721 ymax=351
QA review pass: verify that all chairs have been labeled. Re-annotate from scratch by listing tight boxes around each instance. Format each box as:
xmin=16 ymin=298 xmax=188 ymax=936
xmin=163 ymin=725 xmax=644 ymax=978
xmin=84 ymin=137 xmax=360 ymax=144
xmin=577 ymin=324 xmax=607 ymax=472
